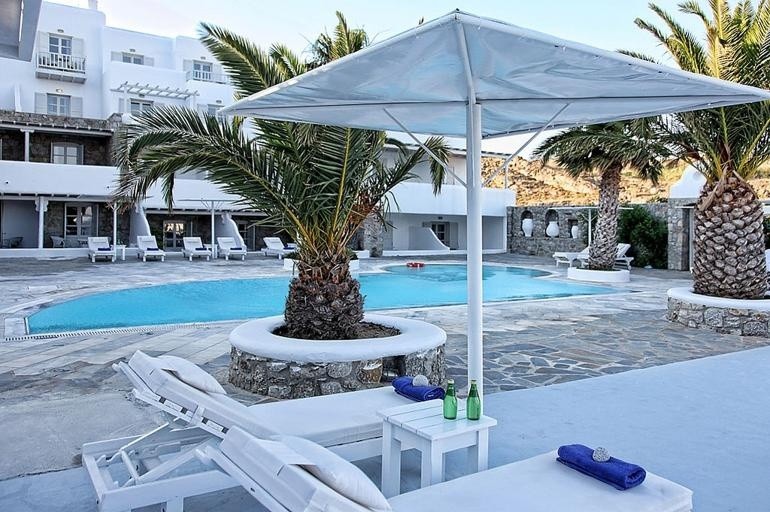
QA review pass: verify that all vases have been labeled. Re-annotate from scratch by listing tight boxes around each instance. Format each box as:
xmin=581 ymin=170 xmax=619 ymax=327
xmin=546 ymin=221 xmax=560 ymax=237
xmin=571 ymin=226 xmax=578 ymax=239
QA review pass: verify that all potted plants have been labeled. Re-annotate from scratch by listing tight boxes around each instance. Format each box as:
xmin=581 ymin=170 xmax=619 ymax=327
xmin=533 ymin=0 xmax=769 ymax=341
xmin=566 ymin=123 xmax=649 ymax=284
xmin=113 ymin=8 xmax=450 ymax=404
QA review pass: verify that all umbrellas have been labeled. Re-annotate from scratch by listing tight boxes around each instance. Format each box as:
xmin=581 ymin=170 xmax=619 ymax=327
xmin=218 ymin=6 xmax=770 ymax=458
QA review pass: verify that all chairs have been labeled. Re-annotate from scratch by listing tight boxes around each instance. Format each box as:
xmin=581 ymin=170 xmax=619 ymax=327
xmin=553 ymin=246 xmax=589 ymax=268
xmin=50 ymin=236 xmax=68 ymax=248
xmin=2 ymin=236 xmax=23 ymax=247
xmin=579 ymin=243 xmax=635 ymax=270
xmin=213 ymin=420 xmax=694 ymax=512
xmin=86 ymin=232 xmax=296 ymax=261
xmin=82 ymin=352 xmax=422 ymax=512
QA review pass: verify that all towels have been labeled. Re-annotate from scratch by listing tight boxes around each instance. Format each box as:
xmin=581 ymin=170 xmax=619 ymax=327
xmin=391 ymin=376 xmax=445 ymax=401
xmin=558 ymin=442 xmax=646 ymax=492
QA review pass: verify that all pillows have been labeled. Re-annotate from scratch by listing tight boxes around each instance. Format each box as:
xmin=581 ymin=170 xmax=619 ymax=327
xmin=157 ymin=356 xmax=227 ymax=397
xmin=269 ymin=434 xmax=392 ymax=511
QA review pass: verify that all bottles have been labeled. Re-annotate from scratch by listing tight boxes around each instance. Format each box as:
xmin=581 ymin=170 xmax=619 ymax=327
xmin=545 ymin=221 xmax=559 ymax=237
xmin=466 ymin=379 xmax=481 ymax=421
xmin=442 ymin=378 xmax=458 ymax=420
xmin=571 ymin=225 xmax=578 ymax=238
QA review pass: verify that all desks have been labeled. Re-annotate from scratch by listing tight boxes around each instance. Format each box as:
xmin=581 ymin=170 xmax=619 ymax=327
xmin=77 ymin=239 xmax=88 ymax=248
xmin=376 ymin=399 xmax=496 ymax=500
